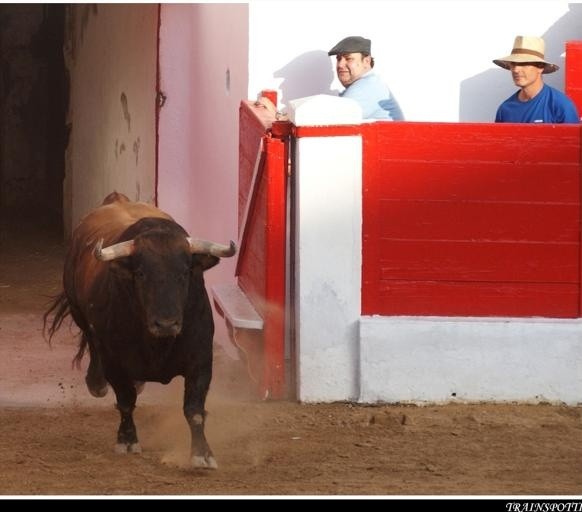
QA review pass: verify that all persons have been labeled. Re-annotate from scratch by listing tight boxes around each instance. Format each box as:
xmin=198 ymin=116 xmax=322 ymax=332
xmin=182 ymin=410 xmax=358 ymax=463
xmin=325 ymin=33 xmax=407 ymax=123
xmin=486 ymin=30 xmax=582 ymax=127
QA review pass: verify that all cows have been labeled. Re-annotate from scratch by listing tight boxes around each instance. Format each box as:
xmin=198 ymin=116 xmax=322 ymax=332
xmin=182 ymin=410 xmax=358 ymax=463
xmin=40 ymin=192 xmax=238 ymax=471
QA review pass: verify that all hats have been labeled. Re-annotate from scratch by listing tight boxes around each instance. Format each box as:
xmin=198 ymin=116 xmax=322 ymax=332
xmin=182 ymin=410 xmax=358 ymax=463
xmin=327 ymin=35 xmax=372 ymax=56
xmin=494 ymin=34 xmax=560 ymax=74
